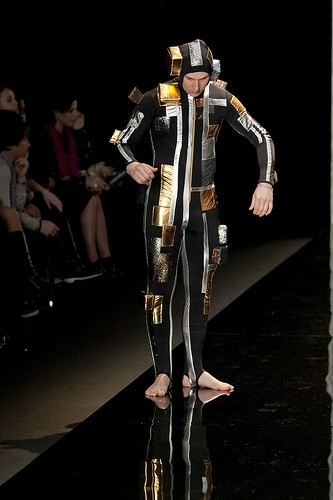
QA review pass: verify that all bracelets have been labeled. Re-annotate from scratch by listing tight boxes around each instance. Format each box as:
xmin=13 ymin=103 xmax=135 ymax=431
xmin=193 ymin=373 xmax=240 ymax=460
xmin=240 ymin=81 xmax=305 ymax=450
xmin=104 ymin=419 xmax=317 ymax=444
xmin=36 ymin=219 xmax=42 ymax=231
xmin=257 ymin=184 xmax=273 ymax=190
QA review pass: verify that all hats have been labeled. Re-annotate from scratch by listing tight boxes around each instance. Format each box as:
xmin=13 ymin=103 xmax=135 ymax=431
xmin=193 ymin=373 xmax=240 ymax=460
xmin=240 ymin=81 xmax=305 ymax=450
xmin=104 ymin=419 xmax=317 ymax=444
xmin=166 ymin=39 xmax=221 ymax=87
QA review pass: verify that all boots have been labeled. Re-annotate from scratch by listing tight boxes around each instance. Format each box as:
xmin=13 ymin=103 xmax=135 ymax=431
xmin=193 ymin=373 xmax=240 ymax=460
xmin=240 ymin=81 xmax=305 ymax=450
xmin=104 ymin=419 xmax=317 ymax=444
xmin=53 ymin=217 xmax=103 ymax=286
xmin=7 ymin=231 xmax=54 ymax=298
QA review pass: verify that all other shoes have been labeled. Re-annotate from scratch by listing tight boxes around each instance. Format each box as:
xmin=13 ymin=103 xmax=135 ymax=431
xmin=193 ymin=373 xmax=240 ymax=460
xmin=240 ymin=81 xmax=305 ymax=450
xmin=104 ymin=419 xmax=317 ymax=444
xmin=90 ymin=261 xmax=131 ymax=281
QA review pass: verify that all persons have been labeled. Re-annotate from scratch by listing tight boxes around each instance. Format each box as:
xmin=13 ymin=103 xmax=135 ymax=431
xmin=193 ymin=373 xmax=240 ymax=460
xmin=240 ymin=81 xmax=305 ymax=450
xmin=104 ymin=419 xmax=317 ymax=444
xmin=30 ymin=95 xmax=125 ymax=279
xmin=144 ymin=387 xmax=230 ymax=500
xmin=109 ymin=39 xmax=278 ymax=396
xmin=0 ymin=86 xmax=105 ymax=317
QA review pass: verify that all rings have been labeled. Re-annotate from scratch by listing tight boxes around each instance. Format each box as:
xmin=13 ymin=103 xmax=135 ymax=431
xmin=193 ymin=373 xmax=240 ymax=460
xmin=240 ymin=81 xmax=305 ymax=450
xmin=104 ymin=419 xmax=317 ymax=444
xmin=94 ymin=184 xmax=98 ymax=188
